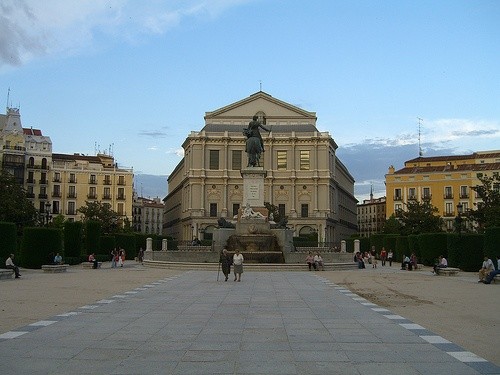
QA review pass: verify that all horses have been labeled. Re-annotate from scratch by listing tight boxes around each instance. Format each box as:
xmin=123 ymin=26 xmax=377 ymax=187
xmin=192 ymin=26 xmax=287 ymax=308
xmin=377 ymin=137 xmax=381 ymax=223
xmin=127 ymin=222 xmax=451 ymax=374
xmin=243 ymin=126 xmax=261 ymax=167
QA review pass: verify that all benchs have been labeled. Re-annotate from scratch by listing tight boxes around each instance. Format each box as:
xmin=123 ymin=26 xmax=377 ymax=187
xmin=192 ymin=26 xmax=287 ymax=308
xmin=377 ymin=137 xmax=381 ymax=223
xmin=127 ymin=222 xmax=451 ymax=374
xmin=406 ymin=263 xmax=424 ymax=271
xmin=0 ymin=269 xmax=14 ymax=280
xmin=42 ymin=264 xmax=69 ymax=274
xmin=434 ymin=267 xmax=460 ymax=277
xmin=479 ymin=270 xmax=500 ymax=284
xmin=80 ymin=262 xmax=102 ymax=269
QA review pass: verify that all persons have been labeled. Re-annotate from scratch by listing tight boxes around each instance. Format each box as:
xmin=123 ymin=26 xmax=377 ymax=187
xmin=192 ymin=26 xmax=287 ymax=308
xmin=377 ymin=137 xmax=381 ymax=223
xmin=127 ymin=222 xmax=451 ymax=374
xmin=219 ymin=249 xmax=232 ymax=281
xmin=89 ymin=253 xmax=97 ymax=269
xmin=138 ymin=248 xmax=144 ymax=263
xmin=54 ymin=253 xmax=62 ymax=264
xmin=409 ymin=253 xmax=417 ymax=270
xmin=387 ymin=249 xmax=393 ymax=266
xmin=354 ymin=252 xmax=366 ymax=269
xmin=232 ymin=249 xmax=244 ymax=282
xmin=245 ymin=115 xmax=270 ymax=152
xmin=314 ymin=252 xmax=325 ymax=271
xmin=242 ymin=203 xmax=263 ymax=217
xmin=192 ymin=238 xmax=201 ymax=246
xmin=401 ymin=254 xmax=410 ymax=270
xmin=432 ymin=255 xmax=447 ymax=275
xmin=380 ymin=247 xmax=386 ymax=266
xmin=5 ymin=253 xmax=21 ymax=278
xmin=478 ymin=256 xmax=500 ymax=284
xmin=365 ymin=250 xmax=377 ymax=268
xmin=306 ymin=252 xmax=317 ymax=271
xmin=110 ymin=247 xmax=125 ymax=268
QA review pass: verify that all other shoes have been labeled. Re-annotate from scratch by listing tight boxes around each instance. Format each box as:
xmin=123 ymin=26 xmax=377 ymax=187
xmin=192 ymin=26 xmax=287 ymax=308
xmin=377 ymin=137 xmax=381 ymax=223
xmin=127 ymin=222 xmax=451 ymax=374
xmin=225 ymin=278 xmax=228 ymax=281
xmin=478 ymin=280 xmax=490 ymax=284
xmin=15 ymin=276 xmax=20 ymax=278
xmin=234 ymin=278 xmax=237 ymax=281
xmin=15 ymin=273 xmax=21 ymax=276
xmin=238 ymin=280 xmax=240 ymax=282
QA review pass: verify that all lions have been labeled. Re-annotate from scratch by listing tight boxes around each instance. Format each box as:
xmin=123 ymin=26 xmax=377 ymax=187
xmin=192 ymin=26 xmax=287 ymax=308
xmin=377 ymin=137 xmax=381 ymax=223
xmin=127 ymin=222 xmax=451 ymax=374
xmin=217 ymin=217 xmax=235 ymax=228
